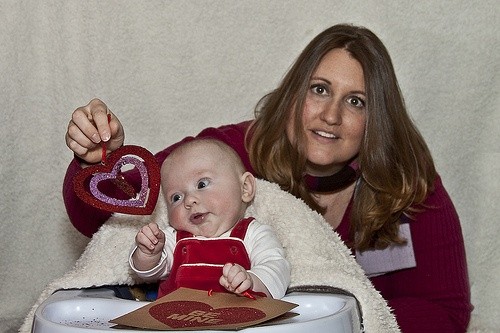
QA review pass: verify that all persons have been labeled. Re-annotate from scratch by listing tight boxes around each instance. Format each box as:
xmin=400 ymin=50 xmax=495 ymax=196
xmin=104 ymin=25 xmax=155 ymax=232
xmin=128 ymin=138 xmax=291 ymax=299
xmin=61 ymin=24 xmax=475 ymax=333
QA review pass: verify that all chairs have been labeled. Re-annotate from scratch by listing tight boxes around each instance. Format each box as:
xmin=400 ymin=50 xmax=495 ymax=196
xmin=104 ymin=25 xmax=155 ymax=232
xmin=18 ymin=178 xmax=402 ymax=333
